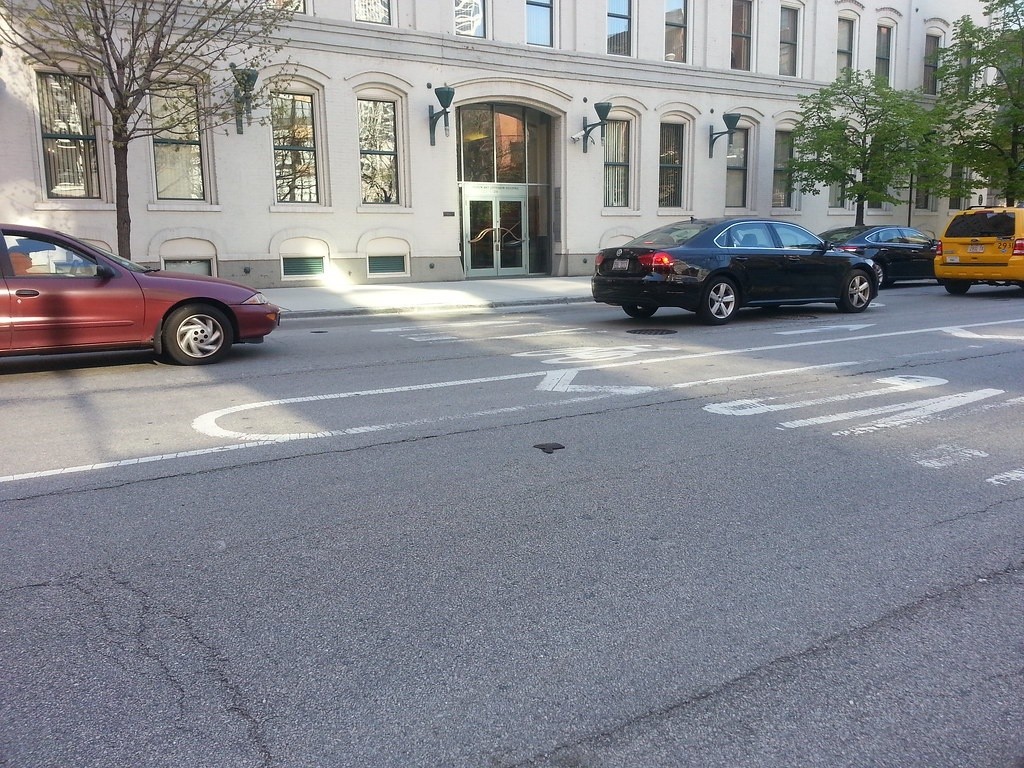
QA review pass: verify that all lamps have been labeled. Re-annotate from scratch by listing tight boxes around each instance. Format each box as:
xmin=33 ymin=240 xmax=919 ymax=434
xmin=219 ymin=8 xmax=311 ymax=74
xmin=582 ymin=102 xmax=612 ymax=152
xmin=232 ymin=69 xmax=259 ymax=134
xmin=709 ymin=113 xmax=741 ymax=159
xmin=428 ymin=86 xmax=454 ymax=146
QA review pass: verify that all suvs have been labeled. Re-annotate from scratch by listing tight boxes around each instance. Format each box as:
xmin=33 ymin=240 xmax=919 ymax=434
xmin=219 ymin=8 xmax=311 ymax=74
xmin=934 ymin=205 xmax=1024 ymax=295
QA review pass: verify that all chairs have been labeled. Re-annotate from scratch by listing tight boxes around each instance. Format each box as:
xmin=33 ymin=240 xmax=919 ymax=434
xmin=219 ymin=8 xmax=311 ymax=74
xmin=740 ymin=234 xmax=758 ymax=248
xmin=882 ymin=232 xmax=894 ymax=243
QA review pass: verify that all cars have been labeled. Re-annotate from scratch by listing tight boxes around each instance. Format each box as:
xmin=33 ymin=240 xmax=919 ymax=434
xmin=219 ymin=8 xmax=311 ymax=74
xmin=0 ymin=223 xmax=282 ymax=376
xmin=591 ymin=214 xmax=879 ymax=326
xmin=816 ymin=224 xmax=939 ymax=286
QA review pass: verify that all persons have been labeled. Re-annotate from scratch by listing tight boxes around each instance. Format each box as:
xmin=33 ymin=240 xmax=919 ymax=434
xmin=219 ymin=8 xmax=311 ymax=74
xmin=8 ymin=246 xmax=32 ymax=275
